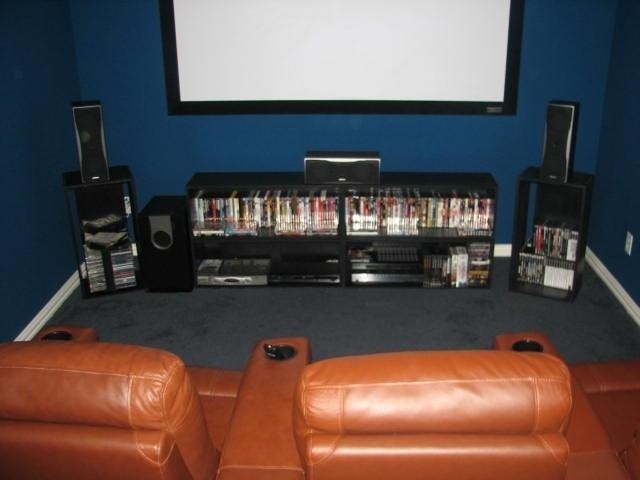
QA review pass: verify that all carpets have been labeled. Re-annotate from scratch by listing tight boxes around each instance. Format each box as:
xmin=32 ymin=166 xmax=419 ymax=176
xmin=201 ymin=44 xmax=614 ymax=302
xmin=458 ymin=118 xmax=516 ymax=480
xmin=43 ymin=257 xmax=640 ymax=369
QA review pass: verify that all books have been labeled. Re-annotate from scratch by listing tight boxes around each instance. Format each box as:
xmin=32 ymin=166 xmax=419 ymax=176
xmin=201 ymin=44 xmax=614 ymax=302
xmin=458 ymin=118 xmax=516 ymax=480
xmin=342 ymin=188 xmax=492 ymax=235
xmin=516 ymin=206 xmax=578 ymax=291
xmin=83 ymin=214 xmax=135 ymax=291
xmin=420 ymin=244 xmax=490 ymax=288
xmin=190 ymin=191 xmax=338 ymax=238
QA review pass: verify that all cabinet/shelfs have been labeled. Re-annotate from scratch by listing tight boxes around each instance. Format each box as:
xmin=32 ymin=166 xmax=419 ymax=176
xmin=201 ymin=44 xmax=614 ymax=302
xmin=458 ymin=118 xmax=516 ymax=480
xmin=508 ymin=176 xmax=595 ymax=304
xmin=186 ymin=184 xmax=498 ymax=289
xmin=64 ymin=178 xmax=149 ymax=297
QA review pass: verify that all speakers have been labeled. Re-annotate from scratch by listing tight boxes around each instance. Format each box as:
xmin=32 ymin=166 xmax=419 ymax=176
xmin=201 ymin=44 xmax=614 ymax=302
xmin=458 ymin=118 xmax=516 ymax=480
xmin=302 ymin=149 xmax=383 ymax=186
xmin=539 ymin=100 xmax=580 ymax=184
xmin=134 ymin=195 xmax=197 ymax=293
xmin=69 ymin=100 xmax=112 ymax=187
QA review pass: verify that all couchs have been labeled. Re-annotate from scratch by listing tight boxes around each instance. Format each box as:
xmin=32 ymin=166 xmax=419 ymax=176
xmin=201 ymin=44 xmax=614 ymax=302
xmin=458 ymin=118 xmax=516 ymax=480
xmin=217 ymin=338 xmax=572 ymax=480
xmin=0 ymin=327 xmax=243 ymax=479
xmin=493 ymin=332 xmax=640 ymax=480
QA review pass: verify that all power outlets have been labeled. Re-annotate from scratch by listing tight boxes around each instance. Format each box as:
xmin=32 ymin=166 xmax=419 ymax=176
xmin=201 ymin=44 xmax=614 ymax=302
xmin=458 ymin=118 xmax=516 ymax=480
xmin=624 ymin=231 xmax=633 ymax=256
